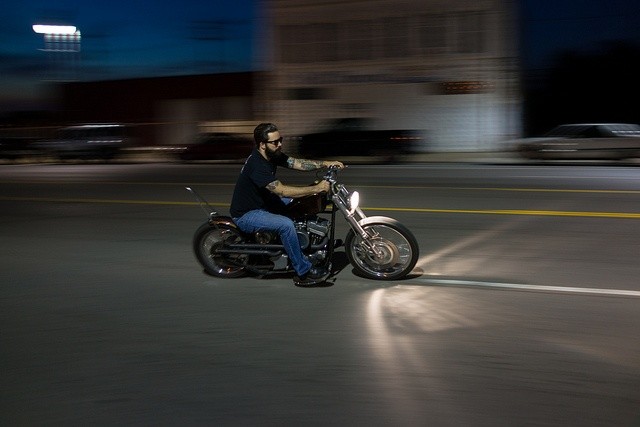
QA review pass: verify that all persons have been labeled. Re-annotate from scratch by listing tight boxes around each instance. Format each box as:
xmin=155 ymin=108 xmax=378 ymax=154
xmin=228 ymin=122 xmax=345 ymax=283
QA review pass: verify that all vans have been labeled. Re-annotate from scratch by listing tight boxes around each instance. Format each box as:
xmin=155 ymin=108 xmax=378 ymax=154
xmin=39 ymin=123 xmax=128 ymax=163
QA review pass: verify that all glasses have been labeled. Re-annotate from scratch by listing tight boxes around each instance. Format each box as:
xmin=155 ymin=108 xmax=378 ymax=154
xmin=267 ymin=137 xmax=282 ymax=145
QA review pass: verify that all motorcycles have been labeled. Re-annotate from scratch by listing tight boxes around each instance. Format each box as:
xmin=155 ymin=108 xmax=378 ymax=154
xmin=184 ymin=161 xmax=421 ymax=284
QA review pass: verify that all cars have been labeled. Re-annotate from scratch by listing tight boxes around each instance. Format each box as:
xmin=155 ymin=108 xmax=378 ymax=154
xmin=521 ymin=121 xmax=639 ymax=162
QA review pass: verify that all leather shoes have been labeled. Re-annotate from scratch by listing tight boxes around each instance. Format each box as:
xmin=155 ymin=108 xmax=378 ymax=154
xmin=299 ymin=265 xmax=329 ymax=282
